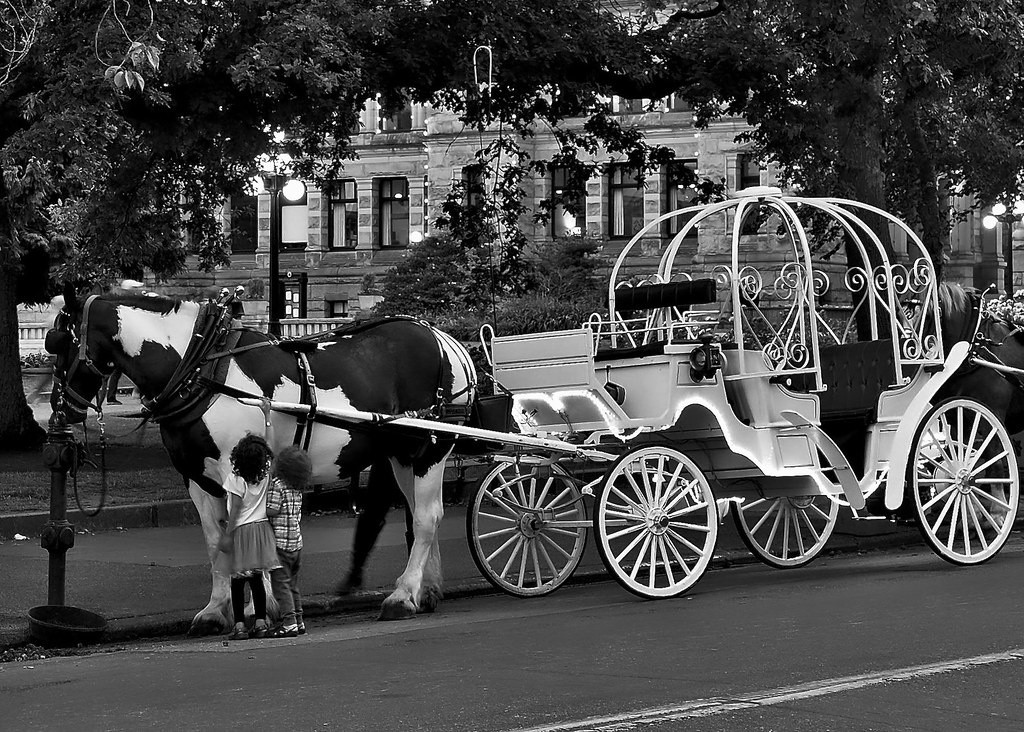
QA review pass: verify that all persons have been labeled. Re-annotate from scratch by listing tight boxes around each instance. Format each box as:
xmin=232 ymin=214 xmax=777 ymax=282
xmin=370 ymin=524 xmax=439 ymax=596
xmin=220 ymin=434 xmax=279 ymax=640
xmin=265 ymin=445 xmax=312 ymax=640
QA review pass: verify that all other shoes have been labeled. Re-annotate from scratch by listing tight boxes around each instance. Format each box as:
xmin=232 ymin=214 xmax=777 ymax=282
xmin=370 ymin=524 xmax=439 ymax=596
xmin=106 ymin=401 xmax=122 ymax=405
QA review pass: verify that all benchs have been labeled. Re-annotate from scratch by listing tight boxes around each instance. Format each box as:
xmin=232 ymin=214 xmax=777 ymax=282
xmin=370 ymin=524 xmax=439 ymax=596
xmin=594 ymin=337 xmax=722 ymax=419
xmin=722 ymin=338 xmax=913 ymax=470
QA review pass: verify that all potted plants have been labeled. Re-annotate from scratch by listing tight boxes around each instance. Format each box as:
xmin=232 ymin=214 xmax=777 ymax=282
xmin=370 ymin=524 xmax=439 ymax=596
xmin=357 ymin=273 xmax=386 ymax=311
xmin=241 ymin=280 xmax=270 ymax=315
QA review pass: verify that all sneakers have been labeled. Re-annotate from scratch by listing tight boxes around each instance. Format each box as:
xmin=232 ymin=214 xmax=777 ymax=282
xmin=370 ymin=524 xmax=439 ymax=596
xmin=266 ymin=622 xmax=306 ymax=637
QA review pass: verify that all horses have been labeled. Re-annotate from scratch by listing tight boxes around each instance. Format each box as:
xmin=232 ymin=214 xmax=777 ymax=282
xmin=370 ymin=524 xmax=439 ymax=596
xmin=45 ymin=279 xmax=477 ymax=638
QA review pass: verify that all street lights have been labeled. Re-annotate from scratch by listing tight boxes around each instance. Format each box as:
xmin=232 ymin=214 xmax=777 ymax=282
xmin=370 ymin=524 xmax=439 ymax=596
xmin=243 ymin=121 xmax=306 ymax=338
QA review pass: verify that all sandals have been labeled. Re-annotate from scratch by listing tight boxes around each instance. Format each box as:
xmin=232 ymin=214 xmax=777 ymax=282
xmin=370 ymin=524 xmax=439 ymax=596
xmin=228 ymin=625 xmax=249 ymax=640
xmin=248 ymin=624 xmax=268 ymax=638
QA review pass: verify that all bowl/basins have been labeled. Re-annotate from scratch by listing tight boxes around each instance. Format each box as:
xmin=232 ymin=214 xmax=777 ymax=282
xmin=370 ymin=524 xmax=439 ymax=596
xmin=27 ymin=606 xmax=108 ymax=646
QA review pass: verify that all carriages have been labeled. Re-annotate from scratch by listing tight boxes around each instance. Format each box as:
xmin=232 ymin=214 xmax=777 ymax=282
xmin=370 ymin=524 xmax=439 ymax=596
xmin=44 ymin=185 xmax=1021 ymax=637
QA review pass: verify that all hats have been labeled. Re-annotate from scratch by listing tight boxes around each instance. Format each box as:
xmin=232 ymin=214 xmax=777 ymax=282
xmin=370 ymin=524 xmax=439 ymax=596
xmin=121 ymin=279 xmax=144 ymax=290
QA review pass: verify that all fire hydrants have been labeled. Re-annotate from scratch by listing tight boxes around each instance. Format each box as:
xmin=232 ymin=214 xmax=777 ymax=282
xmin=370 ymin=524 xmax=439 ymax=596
xmin=38 ymin=410 xmax=82 ymax=604
